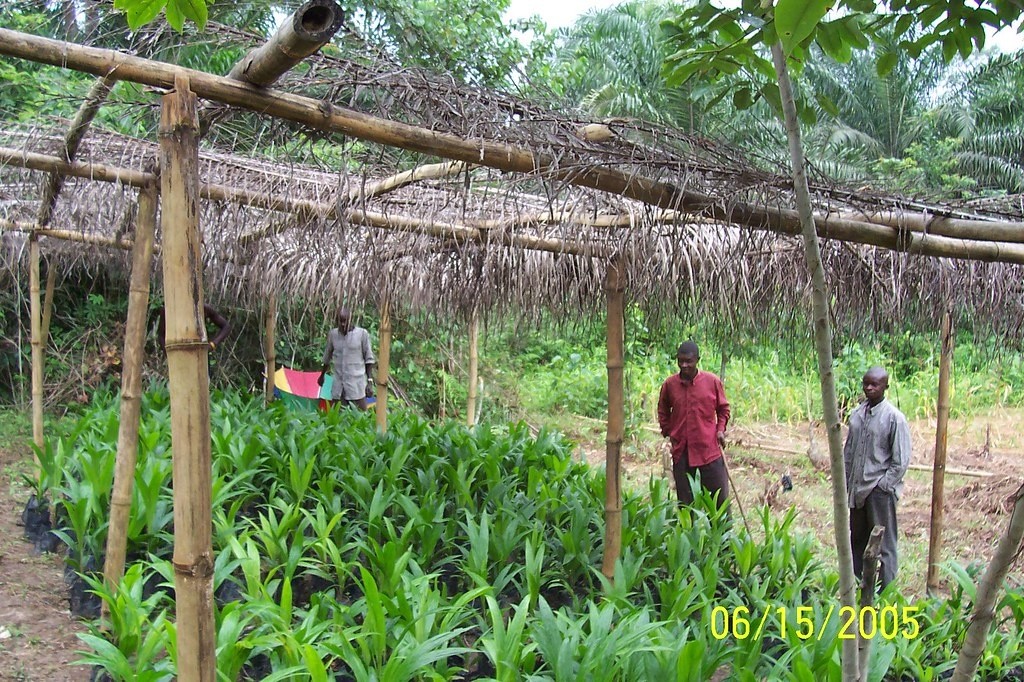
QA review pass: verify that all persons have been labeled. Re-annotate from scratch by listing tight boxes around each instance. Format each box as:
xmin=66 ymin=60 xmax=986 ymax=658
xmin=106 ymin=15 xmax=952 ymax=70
xmin=657 ymin=341 xmax=735 ymax=562
xmin=202 ymin=302 xmax=231 ymax=383
xmin=842 ymin=366 xmax=911 ymax=595
xmin=318 ymin=308 xmax=375 ymax=411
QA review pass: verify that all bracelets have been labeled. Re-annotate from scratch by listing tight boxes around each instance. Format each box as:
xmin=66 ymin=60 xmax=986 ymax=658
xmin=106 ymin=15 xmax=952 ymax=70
xmin=210 ymin=342 xmax=216 ymax=350
xmin=368 ymin=379 xmax=373 ymax=381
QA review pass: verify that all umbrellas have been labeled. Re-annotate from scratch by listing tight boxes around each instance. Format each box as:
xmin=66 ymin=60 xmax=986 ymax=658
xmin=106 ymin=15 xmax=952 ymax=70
xmin=262 ymin=366 xmax=378 ymax=412
